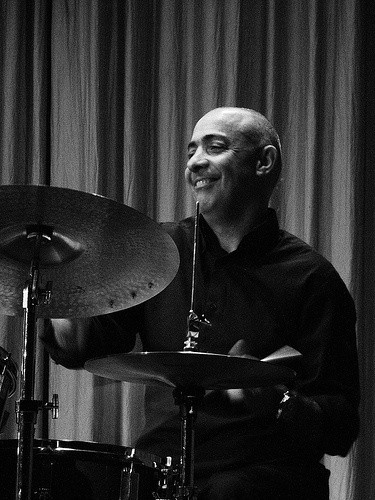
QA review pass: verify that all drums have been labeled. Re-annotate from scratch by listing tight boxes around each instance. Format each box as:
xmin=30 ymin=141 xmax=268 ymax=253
xmin=0 ymin=439 xmax=183 ymax=500
xmin=1 ymin=346 xmax=19 ymax=400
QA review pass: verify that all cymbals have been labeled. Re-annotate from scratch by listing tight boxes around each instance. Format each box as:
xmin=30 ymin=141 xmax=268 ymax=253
xmin=0 ymin=184 xmax=181 ymax=319
xmin=124 ymin=351 xmax=272 ymax=380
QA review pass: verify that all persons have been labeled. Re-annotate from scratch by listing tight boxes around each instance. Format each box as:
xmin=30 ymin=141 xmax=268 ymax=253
xmin=45 ymin=106 xmax=361 ymax=500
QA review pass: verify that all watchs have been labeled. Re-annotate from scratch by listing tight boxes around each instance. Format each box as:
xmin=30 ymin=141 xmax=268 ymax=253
xmin=276 ymin=385 xmax=298 ymax=423
